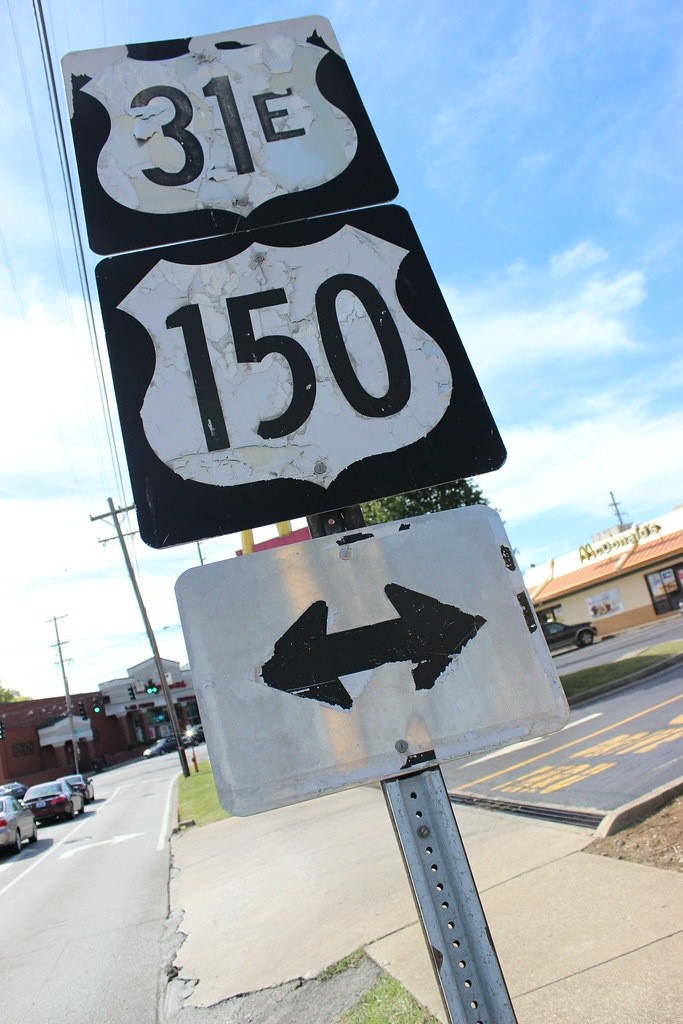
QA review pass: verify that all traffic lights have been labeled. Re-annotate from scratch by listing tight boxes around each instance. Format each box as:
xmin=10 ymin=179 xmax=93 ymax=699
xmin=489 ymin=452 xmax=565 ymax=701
xmin=145 ymin=687 xmax=158 ymax=694
xmin=102 ymin=696 xmax=110 ymax=703
xmin=79 ymin=702 xmax=87 ymax=721
xmin=128 ymin=686 xmax=136 ymax=700
xmin=93 ymin=706 xmax=101 ymax=713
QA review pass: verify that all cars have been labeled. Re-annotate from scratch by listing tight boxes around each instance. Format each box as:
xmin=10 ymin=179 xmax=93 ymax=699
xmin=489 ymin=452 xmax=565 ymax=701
xmin=0 ymin=795 xmax=37 ymax=855
xmin=56 ymin=774 xmax=95 ymax=805
xmin=180 ymin=724 xmax=205 ymax=749
xmin=143 ymin=737 xmax=178 ymax=758
xmin=541 ymin=622 xmax=597 ymax=652
xmin=0 ymin=782 xmax=29 ymax=800
xmin=21 ymin=780 xmax=85 ymax=823
